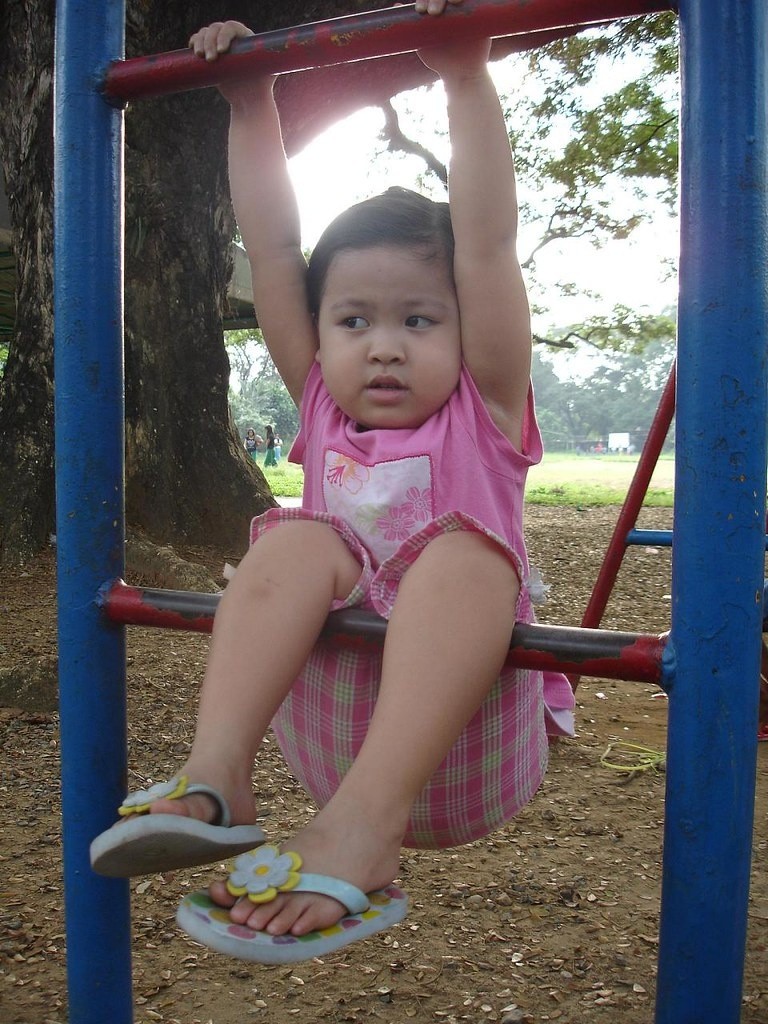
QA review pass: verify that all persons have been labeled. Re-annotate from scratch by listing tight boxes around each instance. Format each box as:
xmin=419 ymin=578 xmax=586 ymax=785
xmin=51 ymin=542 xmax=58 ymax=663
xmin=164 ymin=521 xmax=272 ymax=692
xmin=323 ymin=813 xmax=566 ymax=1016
xmin=90 ymin=0 xmax=576 ymax=965
xmin=264 ymin=424 xmax=282 ymax=468
xmin=244 ymin=428 xmax=261 ymax=462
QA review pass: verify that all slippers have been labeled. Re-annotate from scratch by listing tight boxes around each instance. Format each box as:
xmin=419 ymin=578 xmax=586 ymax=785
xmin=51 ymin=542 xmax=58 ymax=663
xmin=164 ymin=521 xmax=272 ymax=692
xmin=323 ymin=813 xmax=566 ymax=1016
xmin=177 ymin=842 xmax=409 ymax=963
xmin=89 ymin=775 xmax=264 ymax=878
